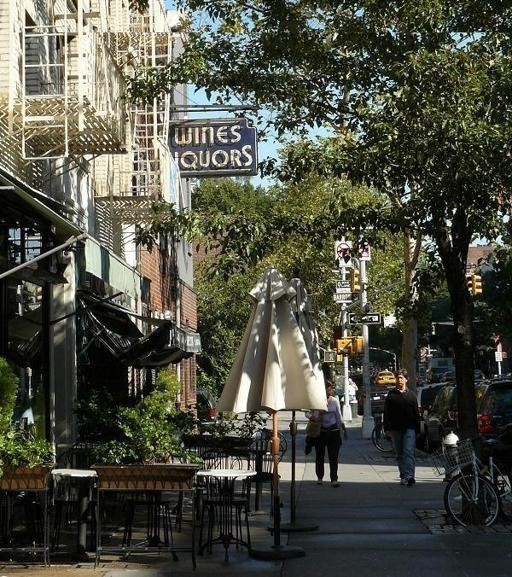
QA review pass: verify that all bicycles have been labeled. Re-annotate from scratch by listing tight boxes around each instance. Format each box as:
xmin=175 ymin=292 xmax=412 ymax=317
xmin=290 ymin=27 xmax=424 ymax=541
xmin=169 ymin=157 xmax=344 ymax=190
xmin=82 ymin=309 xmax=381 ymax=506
xmin=371 ymin=422 xmax=396 ymax=452
xmin=432 ymin=448 xmax=512 ymax=527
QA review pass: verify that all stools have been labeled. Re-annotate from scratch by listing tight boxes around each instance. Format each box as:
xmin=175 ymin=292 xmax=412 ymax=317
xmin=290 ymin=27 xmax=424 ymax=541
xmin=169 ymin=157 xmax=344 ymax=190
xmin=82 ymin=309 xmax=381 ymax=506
xmin=49 ymin=467 xmax=102 ymax=569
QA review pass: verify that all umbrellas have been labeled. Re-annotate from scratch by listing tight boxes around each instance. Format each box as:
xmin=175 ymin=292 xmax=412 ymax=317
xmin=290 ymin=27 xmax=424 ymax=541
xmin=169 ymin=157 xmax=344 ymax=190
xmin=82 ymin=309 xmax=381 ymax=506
xmin=216 ymin=265 xmax=329 ymax=547
xmin=286 ymin=277 xmax=320 ymax=526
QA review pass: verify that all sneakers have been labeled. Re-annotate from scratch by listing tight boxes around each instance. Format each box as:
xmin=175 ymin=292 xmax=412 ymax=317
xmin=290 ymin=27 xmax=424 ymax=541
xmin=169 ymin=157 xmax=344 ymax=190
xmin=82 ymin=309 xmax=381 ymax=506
xmin=331 ymin=480 xmax=339 ymax=487
xmin=317 ymin=478 xmax=323 ymax=484
xmin=400 ymin=477 xmax=415 ymax=485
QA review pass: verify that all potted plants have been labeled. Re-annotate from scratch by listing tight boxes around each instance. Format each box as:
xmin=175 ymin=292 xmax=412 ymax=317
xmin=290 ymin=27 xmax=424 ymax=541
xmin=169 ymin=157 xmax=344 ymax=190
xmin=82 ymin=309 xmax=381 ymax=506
xmin=91 ymin=366 xmax=204 ymax=491
xmin=0 ymin=407 xmax=58 ymax=492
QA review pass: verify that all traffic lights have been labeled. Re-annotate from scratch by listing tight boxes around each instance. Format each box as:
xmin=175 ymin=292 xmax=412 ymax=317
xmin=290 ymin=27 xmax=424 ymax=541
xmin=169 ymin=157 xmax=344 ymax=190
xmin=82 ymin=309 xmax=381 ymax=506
xmin=431 ymin=325 xmax=436 ymax=335
xmin=350 ymin=269 xmax=360 ymax=292
xmin=466 ymin=275 xmax=482 ymax=294
xmin=336 ymin=335 xmax=365 ymax=356
xmin=324 ymin=351 xmax=336 ymax=362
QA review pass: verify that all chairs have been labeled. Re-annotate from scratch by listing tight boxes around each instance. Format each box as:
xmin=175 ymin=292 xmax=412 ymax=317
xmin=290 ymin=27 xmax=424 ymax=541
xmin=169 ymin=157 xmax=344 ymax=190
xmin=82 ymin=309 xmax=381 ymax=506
xmin=121 ymin=427 xmax=288 ymax=560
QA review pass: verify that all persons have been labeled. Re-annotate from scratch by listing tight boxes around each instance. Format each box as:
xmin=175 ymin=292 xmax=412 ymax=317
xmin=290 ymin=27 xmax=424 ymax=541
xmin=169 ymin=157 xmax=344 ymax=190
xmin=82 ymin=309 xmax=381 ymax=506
xmin=305 ymin=379 xmax=349 ymax=488
xmin=383 ymin=368 xmax=421 ymax=488
xmin=348 ymin=378 xmax=359 ymax=403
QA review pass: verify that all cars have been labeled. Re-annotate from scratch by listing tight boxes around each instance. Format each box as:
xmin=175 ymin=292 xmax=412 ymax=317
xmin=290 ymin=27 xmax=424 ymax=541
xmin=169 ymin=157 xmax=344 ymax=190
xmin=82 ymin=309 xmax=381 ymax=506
xmin=355 ymin=384 xmax=389 ymax=415
xmin=372 ymin=371 xmax=396 ymax=386
xmin=196 ymin=390 xmax=218 ymax=422
xmin=417 ymin=366 xmax=512 ymax=454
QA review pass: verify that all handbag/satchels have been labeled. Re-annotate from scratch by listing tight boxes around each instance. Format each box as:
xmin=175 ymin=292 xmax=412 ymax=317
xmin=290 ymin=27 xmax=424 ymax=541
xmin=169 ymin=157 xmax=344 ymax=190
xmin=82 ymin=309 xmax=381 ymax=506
xmin=306 ymin=421 xmax=321 ymax=437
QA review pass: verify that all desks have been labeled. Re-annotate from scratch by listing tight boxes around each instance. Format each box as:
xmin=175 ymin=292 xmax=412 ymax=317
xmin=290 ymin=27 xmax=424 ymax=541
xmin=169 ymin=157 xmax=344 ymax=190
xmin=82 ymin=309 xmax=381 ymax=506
xmin=195 ymin=467 xmax=257 ymax=564
